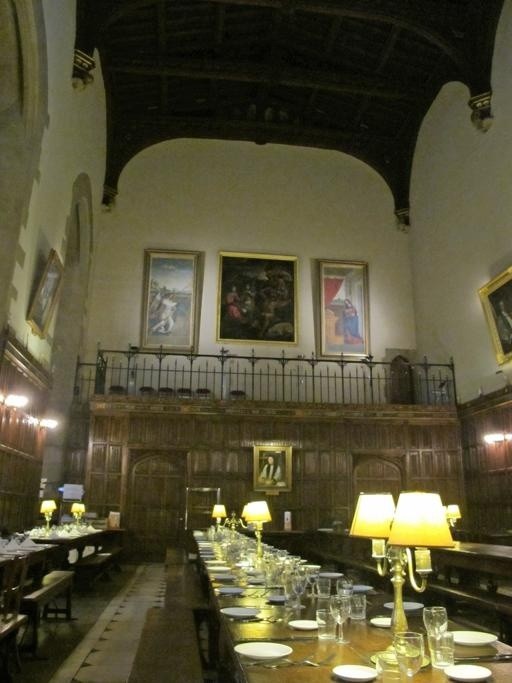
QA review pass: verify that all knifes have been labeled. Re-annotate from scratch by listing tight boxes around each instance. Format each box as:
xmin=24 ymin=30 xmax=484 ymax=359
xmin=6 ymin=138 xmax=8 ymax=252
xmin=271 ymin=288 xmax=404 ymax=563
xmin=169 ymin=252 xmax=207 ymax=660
xmin=237 ymin=635 xmax=317 ymax=643
xmin=455 ymin=654 xmax=512 ymax=664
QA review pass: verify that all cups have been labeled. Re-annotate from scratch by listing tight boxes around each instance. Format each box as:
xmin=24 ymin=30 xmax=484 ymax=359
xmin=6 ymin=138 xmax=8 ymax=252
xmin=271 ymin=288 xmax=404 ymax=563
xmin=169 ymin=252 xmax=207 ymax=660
xmin=305 ymin=568 xmax=319 ymax=597
xmin=338 ymin=580 xmax=355 ymax=599
xmin=330 ymin=595 xmax=350 ymax=644
xmin=316 ymin=608 xmax=337 ymax=640
xmin=316 ymin=579 xmax=333 ymax=599
xmin=277 ymin=549 xmax=288 ymax=563
xmin=349 ymin=594 xmax=367 ymax=620
xmin=395 ymin=632 xmax=423 ymax=683
xmin=423 ymin=607 xmax=448 ymax=658
xmin=376 ymin=651 xmax=402 ymax=683
xmin=428 ymin=631 xmax=454 ymax=669
xmin=292 ymin=578 xmax=308 ymax=609
xmin=283 ymin=584 xmax=297 ymax=608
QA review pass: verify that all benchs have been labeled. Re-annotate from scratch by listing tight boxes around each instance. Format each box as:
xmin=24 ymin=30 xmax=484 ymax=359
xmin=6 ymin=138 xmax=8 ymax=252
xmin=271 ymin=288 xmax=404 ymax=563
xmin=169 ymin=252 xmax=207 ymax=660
xmin=166 ymin=564 xmax=210 ymax=671
xmin=0 ymin=611 xmax=28 ymax=674
xmin=405 ymin=575 xmax=512 ymax=643
xmin=166 ymin=547 xmax=189 ymax=567
xmin=127 ymin=608 xmax=203 ymax=682
xmin=21 ymin=570 xmax=74 ymax=662
xmin=70 ymin=542 xmax=128 ymax=594
xmin=310 ymin=546 xmax=391 ymax=592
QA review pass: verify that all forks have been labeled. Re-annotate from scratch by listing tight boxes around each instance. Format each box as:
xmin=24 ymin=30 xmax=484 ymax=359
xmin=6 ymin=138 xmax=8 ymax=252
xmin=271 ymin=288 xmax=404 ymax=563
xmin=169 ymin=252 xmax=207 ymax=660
xmin=242 ymin=652 xmax=338 ymax=669
xmin=238 ymin=613 xmax=283 ymax=626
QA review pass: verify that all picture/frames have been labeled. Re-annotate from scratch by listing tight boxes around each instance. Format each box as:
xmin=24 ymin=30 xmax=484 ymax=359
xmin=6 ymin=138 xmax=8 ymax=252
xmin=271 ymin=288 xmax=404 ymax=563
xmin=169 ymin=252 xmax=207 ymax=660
xmin=253 ymin=445 xmax=293 ymax=495
xmin=140 ymin=249 xmax=205 ymax=360
xmin=215 ymin=250 xmax=299 ymax=348
xmin=26 ymin=248 xmax=65 ymax=340
xmin=477 ymin=265 xmax=512 ymax=365
xmin=314 ymin=258 xmax=371 ymax=363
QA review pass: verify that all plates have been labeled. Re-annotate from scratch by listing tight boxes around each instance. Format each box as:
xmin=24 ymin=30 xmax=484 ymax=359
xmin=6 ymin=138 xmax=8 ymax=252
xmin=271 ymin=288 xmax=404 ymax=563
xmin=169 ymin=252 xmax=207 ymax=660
xmin=248 ymin=578 xmax=266 ymax=584
xmin=214 ymin=575 xmax=237 ymax=582
xmin=444 ymin=664 xmax=492 ymax=682
xmin=353 ymin=584 xmax=373 ymax=592
xmin=332 ymin=664 xmax=377 ymax=682
xmin=235 ymin=561 xmax=257 ymax=566
xmin=268 ymin=595 xmax=285 ymax=603
xmin=214 ymin=587 xmax=246 ymax=595
xmin=370 ymin=653 xmax=431 ymax=668
xmin=288 ymin=619 xmax=318 ymax=631
xmin=319 ymin=572 xmax=344 ymax=578
xmin=371 ymin=616 xmax=392 ymax=628
xmin=219 ymin=607 xmax=261 ymax=618
xmin=385 ymin=601 xmax=424 ymax=612
xmin=235 ymin=642 xmax=294 ymax=659
xmin=301 ymin=564 xmax=322 ymax=571
xmin=452 ymin=630 xmax=497 ymax=647
xmin=200 ymin=555 xmax=220 ymax=560
xmin=207 ymin=566 xmax=231 ymax=572
xmin=204 ymin=560 xmax=228 ymax=565
xmin=301 ymin=559 xmax=308 ymax=563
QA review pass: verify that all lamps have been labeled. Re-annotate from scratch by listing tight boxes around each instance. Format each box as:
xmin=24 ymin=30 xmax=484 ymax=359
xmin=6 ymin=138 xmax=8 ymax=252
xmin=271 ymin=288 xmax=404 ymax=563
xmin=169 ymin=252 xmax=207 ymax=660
xmin=444 ymin=503 xmax=462 ymax=527
xmin=40 ymin=500 xmax=57 ymax=534
xmin=350 ymin=492 xmax=455 ymax=671
xmin=242 ymin=501 xmax=271 ymax=570
xmin=71 ymin=503 xmax=85 ymax=527
xmin=211 ymin=504 xmax=227 ymax=526
